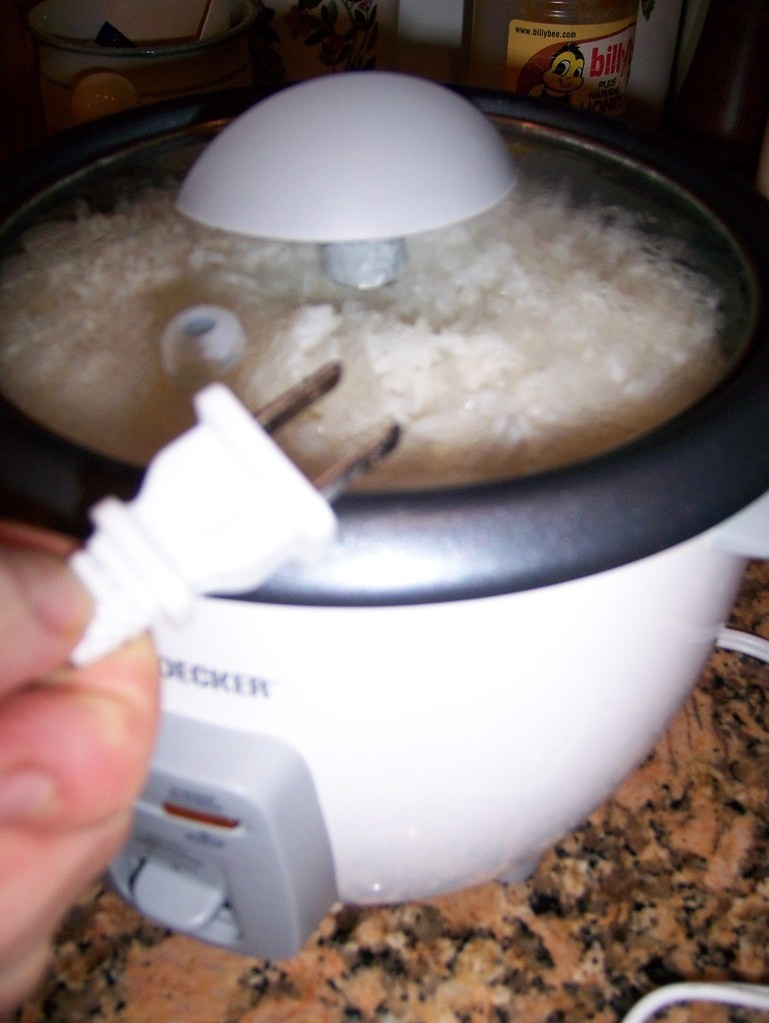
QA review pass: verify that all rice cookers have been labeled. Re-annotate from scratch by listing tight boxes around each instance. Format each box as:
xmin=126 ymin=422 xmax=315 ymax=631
xmin=0 ymin=74 xmax=769 ymax=956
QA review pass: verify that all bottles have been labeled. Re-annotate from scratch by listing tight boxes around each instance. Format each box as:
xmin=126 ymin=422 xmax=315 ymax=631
xmin=471 ymin=0 xmax=636 ymax=120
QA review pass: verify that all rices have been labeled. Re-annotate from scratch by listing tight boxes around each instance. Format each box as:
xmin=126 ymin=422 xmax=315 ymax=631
xmin=1 ymin=176 xmax=722 ymax=493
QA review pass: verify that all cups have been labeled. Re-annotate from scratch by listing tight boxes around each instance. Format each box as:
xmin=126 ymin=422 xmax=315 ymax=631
xmin=27 ymin=0 xmax=256 ymax=135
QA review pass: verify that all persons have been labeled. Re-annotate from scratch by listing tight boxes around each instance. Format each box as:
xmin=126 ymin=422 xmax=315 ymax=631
xmin=0 ymin=526 xmax=162 ymax=1010
